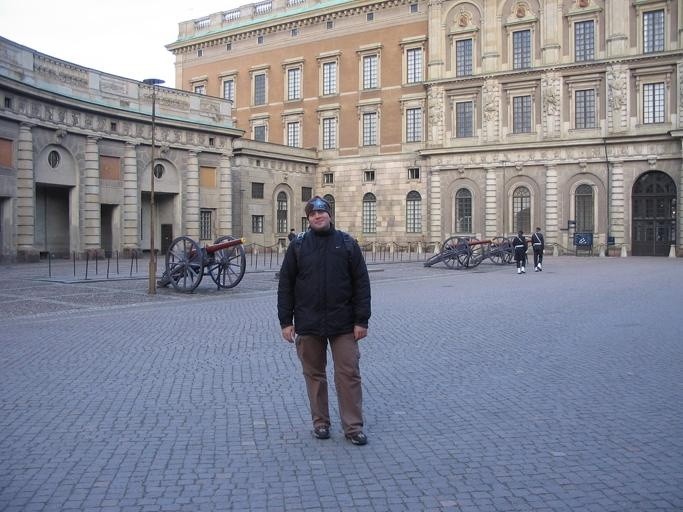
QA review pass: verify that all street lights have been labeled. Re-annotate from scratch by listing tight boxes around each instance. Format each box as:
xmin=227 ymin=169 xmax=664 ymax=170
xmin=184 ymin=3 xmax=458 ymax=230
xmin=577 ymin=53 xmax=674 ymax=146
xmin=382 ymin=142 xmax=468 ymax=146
xmin=142 ymin=79 xmax=166 ymax=295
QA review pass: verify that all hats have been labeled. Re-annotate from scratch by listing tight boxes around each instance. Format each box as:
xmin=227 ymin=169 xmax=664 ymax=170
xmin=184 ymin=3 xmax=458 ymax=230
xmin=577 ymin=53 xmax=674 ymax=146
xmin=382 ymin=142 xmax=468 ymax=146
xmin=305 ymin=196 xmax=332 ymax=218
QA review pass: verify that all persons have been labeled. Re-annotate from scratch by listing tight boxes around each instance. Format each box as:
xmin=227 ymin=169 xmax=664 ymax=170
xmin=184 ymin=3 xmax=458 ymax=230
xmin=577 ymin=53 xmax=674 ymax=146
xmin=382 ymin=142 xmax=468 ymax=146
xmin=512 ymin=227 xmax=545 ymax=274
xmin=288 ymin=228 xmax=298 ymax=241
xmin=277 ymin=195 xmax=372 ymax=446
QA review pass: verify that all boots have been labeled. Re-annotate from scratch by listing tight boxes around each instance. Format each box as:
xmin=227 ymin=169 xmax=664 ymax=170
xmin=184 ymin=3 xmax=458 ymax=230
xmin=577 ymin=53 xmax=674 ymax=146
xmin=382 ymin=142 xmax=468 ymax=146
xmin=534 ymin=263 xmax=543 ymax=272
xmin=517 ymin=266 xmax=524 ymax=273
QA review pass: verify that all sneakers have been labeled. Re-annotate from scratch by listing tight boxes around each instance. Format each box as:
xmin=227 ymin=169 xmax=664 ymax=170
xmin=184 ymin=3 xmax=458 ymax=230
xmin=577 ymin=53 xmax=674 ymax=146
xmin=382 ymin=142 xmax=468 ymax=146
xmin=345 ymin=431 xmax=367 ymax=445
xmin=313 ymin=427 xmax=330 ymax=439
